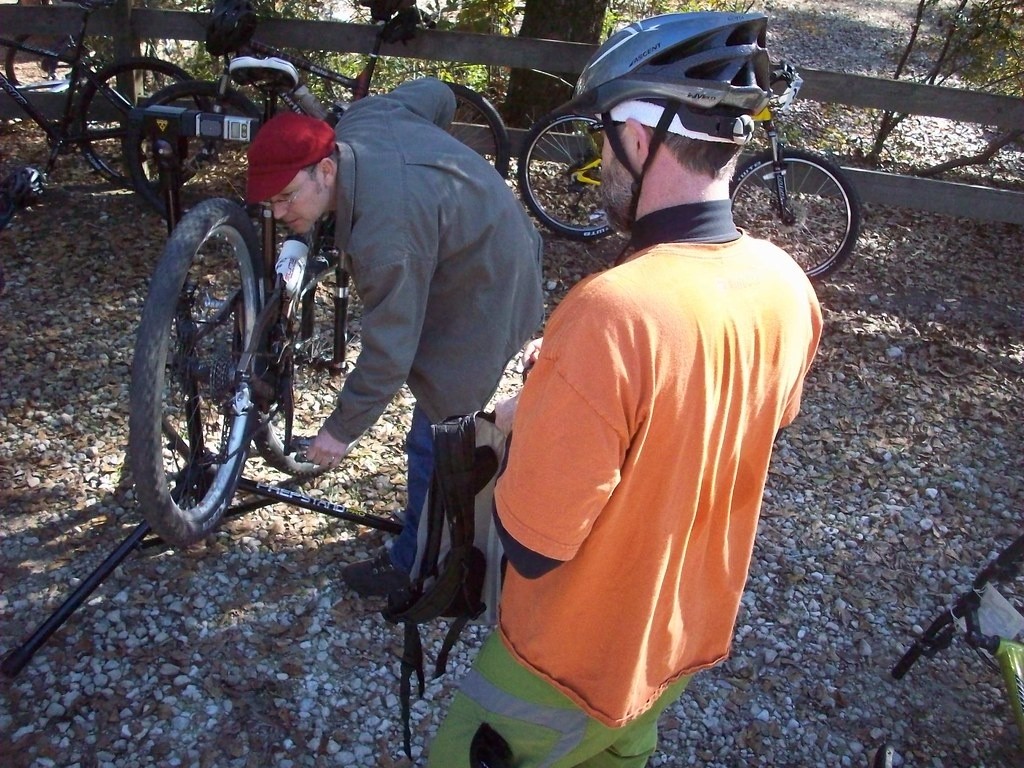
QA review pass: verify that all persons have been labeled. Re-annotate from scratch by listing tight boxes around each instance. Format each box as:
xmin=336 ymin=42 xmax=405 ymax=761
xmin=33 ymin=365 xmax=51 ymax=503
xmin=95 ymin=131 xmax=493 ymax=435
xmin=244 ymin=75 xmax=544 ymax=621
xmin=426 ymin=10 xmax=824 ymax=767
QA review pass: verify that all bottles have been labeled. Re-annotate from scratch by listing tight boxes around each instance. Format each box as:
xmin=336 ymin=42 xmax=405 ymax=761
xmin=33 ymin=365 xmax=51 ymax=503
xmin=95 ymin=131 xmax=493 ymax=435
xmin=275 ymin=235 xmax=309 ymax=303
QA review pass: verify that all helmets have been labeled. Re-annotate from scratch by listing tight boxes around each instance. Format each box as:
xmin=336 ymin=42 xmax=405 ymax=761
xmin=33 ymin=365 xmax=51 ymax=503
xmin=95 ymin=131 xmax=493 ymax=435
xmin=569 ymin=11 xmax=774 ymax=146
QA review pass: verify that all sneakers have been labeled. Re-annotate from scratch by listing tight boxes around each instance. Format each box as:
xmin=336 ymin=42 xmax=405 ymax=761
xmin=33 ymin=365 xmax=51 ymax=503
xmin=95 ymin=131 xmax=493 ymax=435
xmin=341 ymin=547 xmax=411 ymax=595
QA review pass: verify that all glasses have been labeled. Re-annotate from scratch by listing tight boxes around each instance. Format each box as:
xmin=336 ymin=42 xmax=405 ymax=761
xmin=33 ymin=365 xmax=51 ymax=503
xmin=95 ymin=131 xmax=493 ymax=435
xmin=588 ymin=121 xmax=620 ymax=152
xmin=258 ymin=162 xmax=318 ymax=207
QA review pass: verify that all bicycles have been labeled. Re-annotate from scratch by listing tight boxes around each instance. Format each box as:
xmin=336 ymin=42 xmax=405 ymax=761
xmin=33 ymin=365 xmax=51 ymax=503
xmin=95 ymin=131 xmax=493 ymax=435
xmin=120 ymin=0 xmax=511 ymax=224
xmin=517 ymin=60 xmax=858 ymax=283
xmin=1 ymin=0 xmax=215 ymax=195
xmin=128 ymin=55 xmax=370 ymax=550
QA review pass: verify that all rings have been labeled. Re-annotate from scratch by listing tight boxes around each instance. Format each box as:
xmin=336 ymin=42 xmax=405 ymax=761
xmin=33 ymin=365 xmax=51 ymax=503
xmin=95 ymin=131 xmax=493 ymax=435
xmin=328 ymin=456 xmax=334 ymax=463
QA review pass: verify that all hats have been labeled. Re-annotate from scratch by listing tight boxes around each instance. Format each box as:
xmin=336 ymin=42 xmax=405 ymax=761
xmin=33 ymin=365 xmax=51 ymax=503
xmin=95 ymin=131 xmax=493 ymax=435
xmin=246 ymin=110 xmax=336 ymax=204
